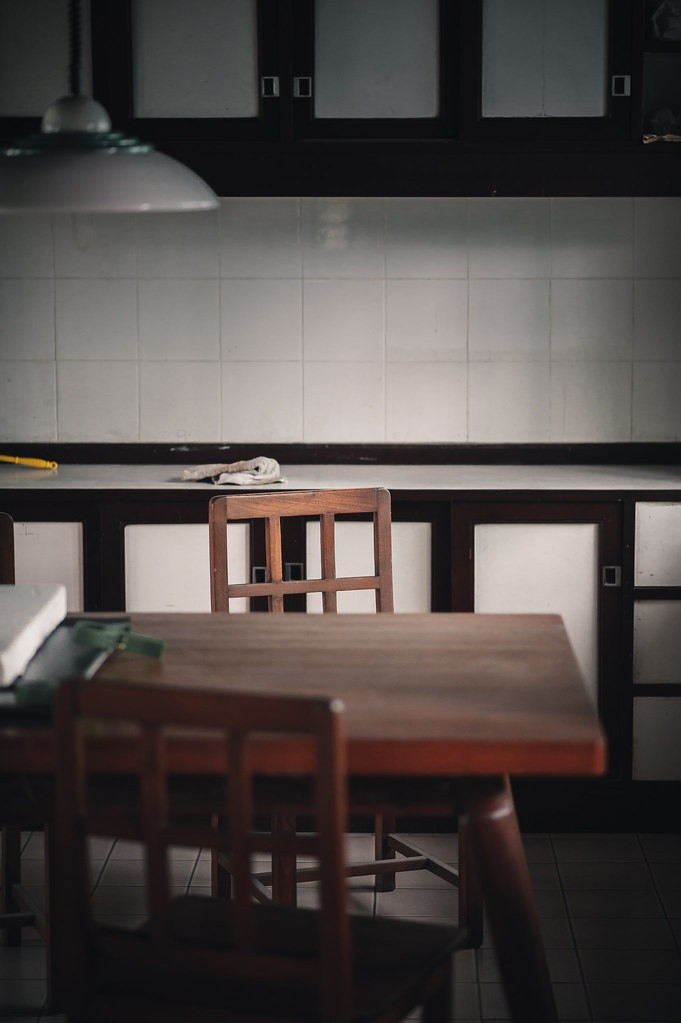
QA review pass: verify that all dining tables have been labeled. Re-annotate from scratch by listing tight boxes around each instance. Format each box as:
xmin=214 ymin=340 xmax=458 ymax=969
xmin=0 ymin=612 xmax=608 ymax=1023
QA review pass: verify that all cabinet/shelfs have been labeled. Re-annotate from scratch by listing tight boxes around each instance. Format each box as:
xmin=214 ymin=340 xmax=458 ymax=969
xmin=0 ymin=489 xmax=96 ymax=831
xmin=452 ymin=491 xmax=624 ymax=833
xmin=631 ymin=0 xmax=681 ymax=197
xmin=621 ymin=490 xmax=680 ymax=834
xmin=110 ymin=0 xmax=461 ymax=197
xmin=461 ymin=0 xmax=631 ymax=197
xmin=97 ymin=491 xmax=452 ymax=833
xmin=0 ymin=0 xmax=110 ymax=149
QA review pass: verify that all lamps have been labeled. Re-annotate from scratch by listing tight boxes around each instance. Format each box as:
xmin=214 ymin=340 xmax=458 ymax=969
xmin=0 ymin=0 xmax=222 ymax=214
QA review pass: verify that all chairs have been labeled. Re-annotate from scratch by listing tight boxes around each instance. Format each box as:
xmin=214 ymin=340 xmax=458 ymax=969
xmin=209 ymin=487 xmax=485 ymax=953
xmin=46 ymin=677 xmax=470 ymax=1023
